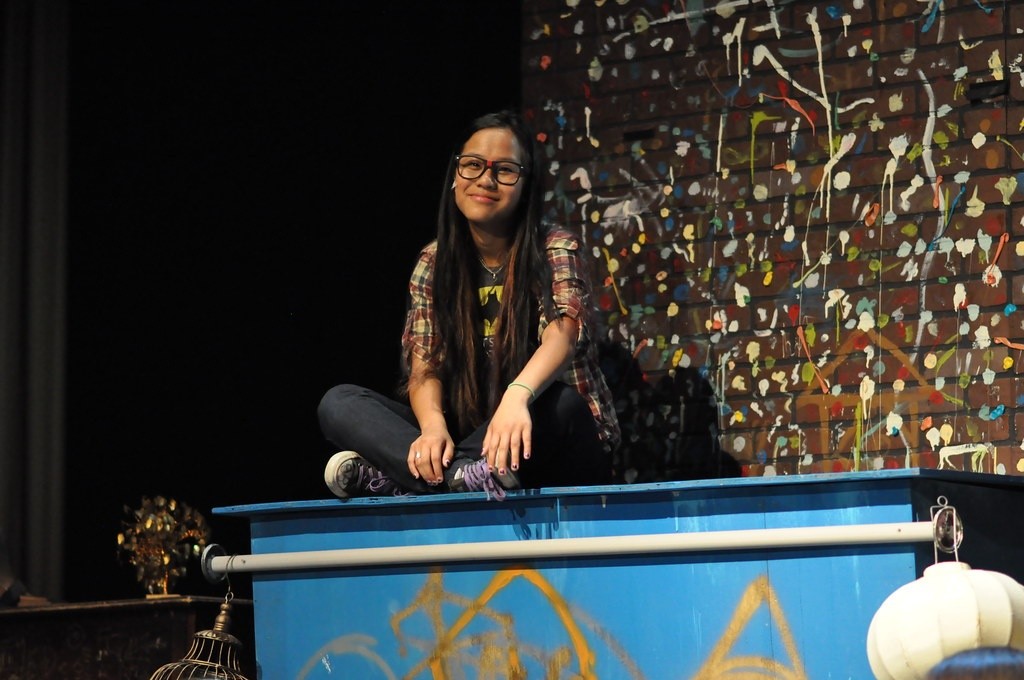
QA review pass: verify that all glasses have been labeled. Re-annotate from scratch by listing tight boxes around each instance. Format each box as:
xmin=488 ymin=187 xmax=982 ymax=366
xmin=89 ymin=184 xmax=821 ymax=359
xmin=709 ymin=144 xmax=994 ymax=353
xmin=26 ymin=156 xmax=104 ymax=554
xmin=456 ymin=155 xmax=528 ymax=185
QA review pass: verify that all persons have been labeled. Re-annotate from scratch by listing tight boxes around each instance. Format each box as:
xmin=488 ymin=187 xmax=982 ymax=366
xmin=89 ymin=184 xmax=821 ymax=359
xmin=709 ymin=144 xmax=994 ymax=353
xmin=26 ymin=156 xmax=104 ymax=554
xmin=318 ymin=113 xmax=621 ymax=498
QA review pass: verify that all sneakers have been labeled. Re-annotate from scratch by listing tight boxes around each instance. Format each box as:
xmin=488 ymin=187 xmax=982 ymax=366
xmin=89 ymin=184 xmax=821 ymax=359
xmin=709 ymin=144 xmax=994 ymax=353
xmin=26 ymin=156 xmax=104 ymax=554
xmin=447 ymin=454 xmax=519 ymax=501
xmin=325 ymin=450 xmax=407 ymax=498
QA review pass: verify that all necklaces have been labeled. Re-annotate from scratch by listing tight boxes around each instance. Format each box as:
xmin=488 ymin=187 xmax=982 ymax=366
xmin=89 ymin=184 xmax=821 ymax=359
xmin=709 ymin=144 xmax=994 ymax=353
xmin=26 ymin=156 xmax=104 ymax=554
xmin=471 ymin=249 xmax=513 ymax=282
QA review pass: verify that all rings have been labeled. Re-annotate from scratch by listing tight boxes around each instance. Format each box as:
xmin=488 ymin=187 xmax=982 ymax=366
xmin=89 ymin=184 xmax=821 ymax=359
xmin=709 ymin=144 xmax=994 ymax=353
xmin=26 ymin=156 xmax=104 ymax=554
xmin=415 ymin=452 xmax=421 ymax=458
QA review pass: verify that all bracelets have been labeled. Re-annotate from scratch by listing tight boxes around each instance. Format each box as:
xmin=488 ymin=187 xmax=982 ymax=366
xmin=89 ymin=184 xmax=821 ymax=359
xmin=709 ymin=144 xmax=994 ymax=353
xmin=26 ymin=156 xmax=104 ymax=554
xmin=505 ymin=382 xmax=536 ymax=404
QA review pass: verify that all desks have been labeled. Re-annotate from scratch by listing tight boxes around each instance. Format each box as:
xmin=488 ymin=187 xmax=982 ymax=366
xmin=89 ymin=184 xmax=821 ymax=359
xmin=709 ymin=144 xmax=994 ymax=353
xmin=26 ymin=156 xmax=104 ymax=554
xmin=211 ymin=466 xmax=1024 ymax=680
